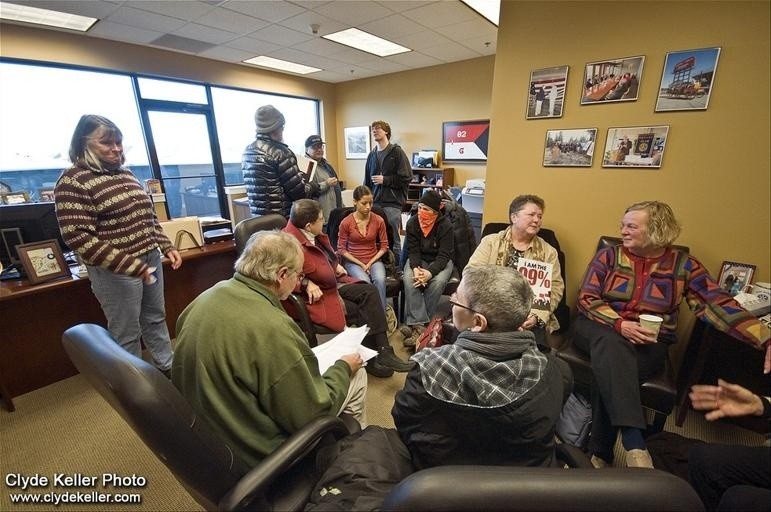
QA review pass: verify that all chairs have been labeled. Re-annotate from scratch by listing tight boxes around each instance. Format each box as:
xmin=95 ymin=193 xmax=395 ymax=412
xmin=228 ymin=214 xmax=344 ymax=349
xmin=436 ymin=220 xmax=572 ymax=362
xmin=402 ymin=195 xmax=480 ymax=327
xmin=557 ymin=232 xmax=692 ymax=455
xmin=52 ymin=318 xmax=365 ymax=511
xmin=324 ymin=206 xmax=402 ymax=325
xmin=377 ymin=462 xmax=704 ymax=512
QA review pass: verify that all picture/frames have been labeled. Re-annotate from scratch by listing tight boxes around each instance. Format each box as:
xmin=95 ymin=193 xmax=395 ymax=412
xmin=654 ymin=46 xmax=722 ymax=114
xmin=577 ymin=52 xmax=647 ymax=106
xmin=13 ymin=237 xmax=74 ymax=286
xmin=602 ymin=125 xmax=673 ymax=169
xmin=523 ymin=64 xmax=569 ymax=120
xmin=715 ymin=257 xmax=757 ymax=299
xmin=343 ymin=126 xmax=371 ymax=160
xmin=542 ymin=126 xmax=600 ymax=168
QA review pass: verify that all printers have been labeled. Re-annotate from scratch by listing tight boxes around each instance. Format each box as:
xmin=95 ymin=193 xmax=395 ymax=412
xmin=461 ymin=179 xmax=486 ymax=214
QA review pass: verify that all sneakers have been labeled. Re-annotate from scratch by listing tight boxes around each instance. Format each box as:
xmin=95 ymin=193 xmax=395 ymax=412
xmin=400 ymin=323 xmax=428 ymax=347
xmin=622 ymin=445 xmax=655 ymax=469
xmin=364 ymin=346 xmax=410 ymax=378
xmin=590 ymin=454 xmax=609 ymax=469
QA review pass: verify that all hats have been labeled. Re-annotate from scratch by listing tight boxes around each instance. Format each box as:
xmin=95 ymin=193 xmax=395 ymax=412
xmin=418 ymin=190 xmax=442 ymax=214
xmin=304 ymin=135 xmax=326 ymax=147
xmin=254 ymin=104 xmax=285 ymax=135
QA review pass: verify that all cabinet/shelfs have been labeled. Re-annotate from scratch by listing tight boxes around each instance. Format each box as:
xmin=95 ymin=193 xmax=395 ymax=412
xmin=403 ymin=164 xmax=456 ymax=210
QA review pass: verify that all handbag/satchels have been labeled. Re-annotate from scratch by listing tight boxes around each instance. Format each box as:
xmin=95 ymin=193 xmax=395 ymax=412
xmin=551 ymin=388 xmax=594 ymax=451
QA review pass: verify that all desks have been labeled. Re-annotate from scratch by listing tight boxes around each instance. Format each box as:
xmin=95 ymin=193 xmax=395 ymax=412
xmin=671 ymin=307 xmax=770 ymax=429
xmin=1 ymin=242 xmax=240 ymax=412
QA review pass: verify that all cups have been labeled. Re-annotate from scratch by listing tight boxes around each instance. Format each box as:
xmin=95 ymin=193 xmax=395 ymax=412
xmin=638 ymin=313 xmax=664 ymax=340
xmin=144 ymin=267 xmax=160 ymax=289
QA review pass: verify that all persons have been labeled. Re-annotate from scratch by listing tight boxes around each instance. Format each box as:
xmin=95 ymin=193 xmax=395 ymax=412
xmin=585 ymin=72 xmax=632 ymax=100
xmin=545 ymin=84 xmax=558 ymax=115
xmin=535 ymin=84 xmax=544 ymax=116
xmin=169 ymin=107 xmax=770 ymax=512
xmin=54 ymin=115 xmax=183 ymax=381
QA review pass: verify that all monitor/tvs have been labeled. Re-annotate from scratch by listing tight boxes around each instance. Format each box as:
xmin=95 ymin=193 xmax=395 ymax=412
xmin=442 ymin=119 xmax=489 ymax=164
xmin=0 ymin=201 xmax=71 ymax=281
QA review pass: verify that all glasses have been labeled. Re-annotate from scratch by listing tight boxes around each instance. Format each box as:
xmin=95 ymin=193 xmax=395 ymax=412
xmin=294 ymin=269 xmax=305 ymax=281
xmin=448 ymin=291 xmax=493 ymax=330
